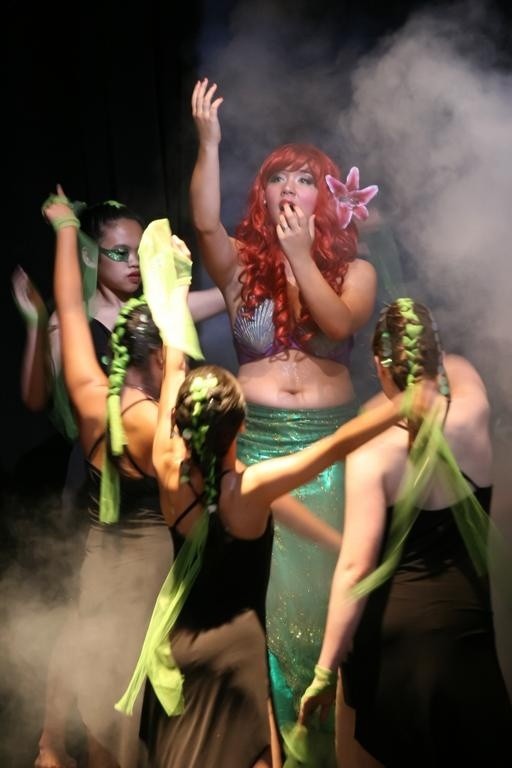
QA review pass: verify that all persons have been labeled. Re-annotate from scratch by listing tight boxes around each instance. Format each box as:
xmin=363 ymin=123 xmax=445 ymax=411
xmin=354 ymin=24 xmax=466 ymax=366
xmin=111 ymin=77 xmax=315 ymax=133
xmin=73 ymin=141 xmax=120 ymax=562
xmin=186 ymin=81 xmax=372 ymax=766
xmin=11 ymin=199 xmax=237 ymax=766
xmin=294 ymin=298 xmax=511 ymax=766
xmin=132 ymin=232 xmax=436 ymax=767
